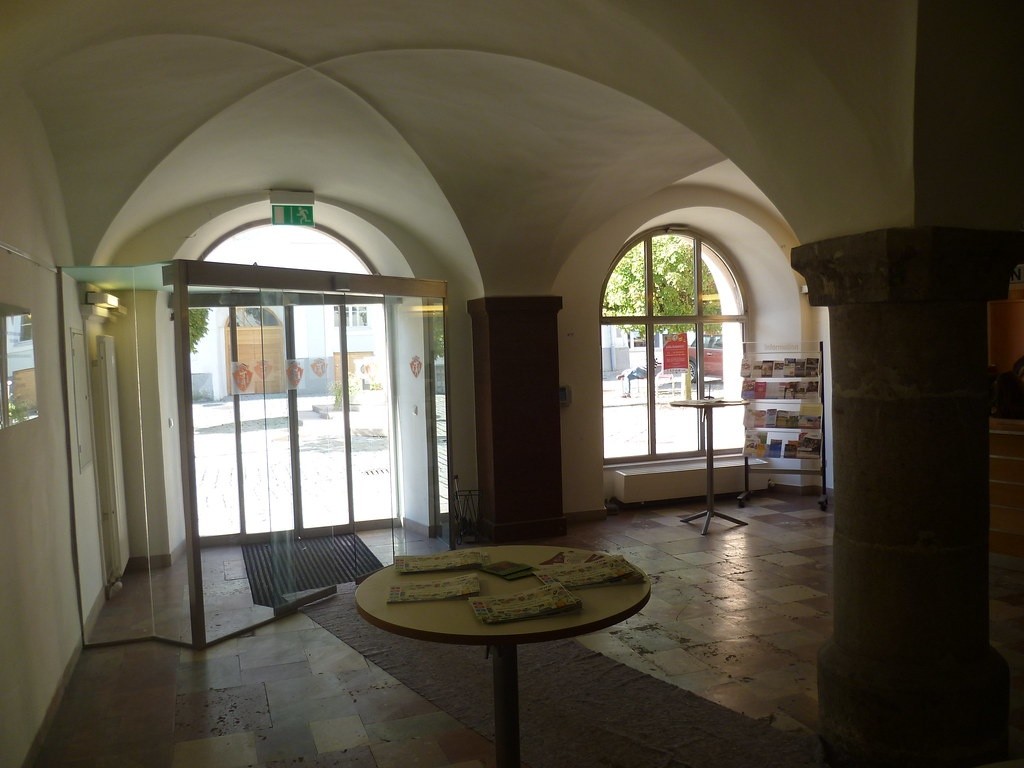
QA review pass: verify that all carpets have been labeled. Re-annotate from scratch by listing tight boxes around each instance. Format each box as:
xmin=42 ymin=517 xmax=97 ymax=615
xmin=241 ymin=533 xmax=384 ymax=609
xmin=284 ymin=581 xmax=866 ymax=768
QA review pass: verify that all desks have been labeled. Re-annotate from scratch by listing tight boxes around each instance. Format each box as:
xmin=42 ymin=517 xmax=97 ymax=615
xmin=670 ymin=399 xmax=751 ymax=535
xmin=356 ymin=545 xmax=651 ymax=768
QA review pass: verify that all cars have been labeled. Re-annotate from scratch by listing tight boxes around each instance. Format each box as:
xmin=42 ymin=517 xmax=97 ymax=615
xmin=688 ymin=333 xmax=723 ymax=383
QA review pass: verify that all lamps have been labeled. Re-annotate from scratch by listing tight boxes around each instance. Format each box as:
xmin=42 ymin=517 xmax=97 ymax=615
xmin=86 ymin=291 xmax=120 ymax=309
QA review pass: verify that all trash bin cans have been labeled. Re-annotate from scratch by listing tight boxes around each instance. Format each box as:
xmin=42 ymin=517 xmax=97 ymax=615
xmin=621 ymin=368 xmax=639 ymax=396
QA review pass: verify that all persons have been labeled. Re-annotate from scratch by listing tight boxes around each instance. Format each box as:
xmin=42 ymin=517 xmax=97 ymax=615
xmin=616 ymin=356 xmax=661 ymax=398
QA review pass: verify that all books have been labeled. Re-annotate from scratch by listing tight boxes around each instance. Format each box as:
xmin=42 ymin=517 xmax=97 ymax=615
xmin=743 ymin=408 xmax=821 ymax=429
xmin=741 ymin=430 xmax=823 ymax=459
xmin=740 ymin=379 xmax=820 ymax=400
xmin=740 ymin=357 xmax=822 ymax=377
